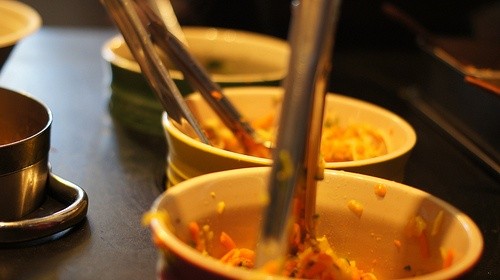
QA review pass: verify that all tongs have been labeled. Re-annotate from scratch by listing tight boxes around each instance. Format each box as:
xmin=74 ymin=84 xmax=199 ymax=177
xmin=100 ymin=0 xmax=272 ymax=159
xmin=261 ymin=2 xmax=340 ymax=268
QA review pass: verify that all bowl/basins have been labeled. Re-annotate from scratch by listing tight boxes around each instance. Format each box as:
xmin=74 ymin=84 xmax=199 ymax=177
xmin=102 ymin=27 xmax=290 ymax=146
xmin=161 ymin=87 xmax=418 ymax=189
xmin=1 ymin=87 xmax=54 ymax=222
xmin=1 ymin=1 xmax=42 ymax=77
xmin=148 ymin=166 xmax=484 ymax=280
